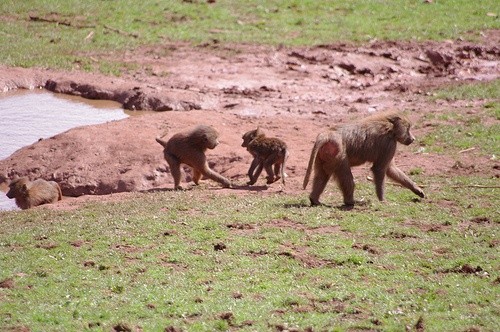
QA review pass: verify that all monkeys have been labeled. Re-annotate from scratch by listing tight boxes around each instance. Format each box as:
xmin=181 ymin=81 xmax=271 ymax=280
xmin=302 ymin=110 xmax=426 ymax=212
xmin=241 ymin=125 xmax=288 ymax=187
xmin=155 ymin=123 xmax=233 ymax=190
